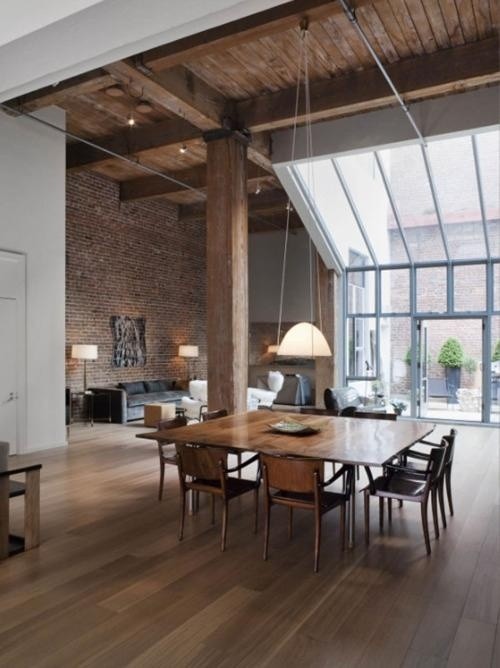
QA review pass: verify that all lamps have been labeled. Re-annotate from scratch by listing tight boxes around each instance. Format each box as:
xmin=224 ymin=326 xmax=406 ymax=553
xmin=71 ymin=344 xmax=98 ymax=394
xmin=179 ymin=344 xmax=199 ymax=386
xmin=275 ymin=16 xmax=335 ymax=358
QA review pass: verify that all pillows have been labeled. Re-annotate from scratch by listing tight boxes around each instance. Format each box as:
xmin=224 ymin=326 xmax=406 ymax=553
xmin=119 ymin=381 xmax=168 ymax=394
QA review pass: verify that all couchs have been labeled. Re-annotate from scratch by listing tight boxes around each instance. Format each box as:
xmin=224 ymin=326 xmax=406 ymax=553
xmin=89 ymin=378 xmax=191 ymax=424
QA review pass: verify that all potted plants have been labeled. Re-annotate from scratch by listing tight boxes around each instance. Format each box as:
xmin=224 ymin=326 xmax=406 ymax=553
xmin=391 ymin=402 xmax=407 ymax=417
xmin=437 ymin=338 xmax=463 ymax=405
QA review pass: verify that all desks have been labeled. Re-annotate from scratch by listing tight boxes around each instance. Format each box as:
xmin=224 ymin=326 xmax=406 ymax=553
xmin=355 ymin=400 xmax=397 ymax=419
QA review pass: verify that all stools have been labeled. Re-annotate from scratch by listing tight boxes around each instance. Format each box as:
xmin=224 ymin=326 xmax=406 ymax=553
xmin=144 ymin=402 xmax=188 ymax=428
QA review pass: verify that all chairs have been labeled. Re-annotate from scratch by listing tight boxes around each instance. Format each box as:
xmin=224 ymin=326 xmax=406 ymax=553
xmin=325 ymin=386 xmax=373 ymax=418
xmin=182 ymin=369 xmax=285 ymax=422
